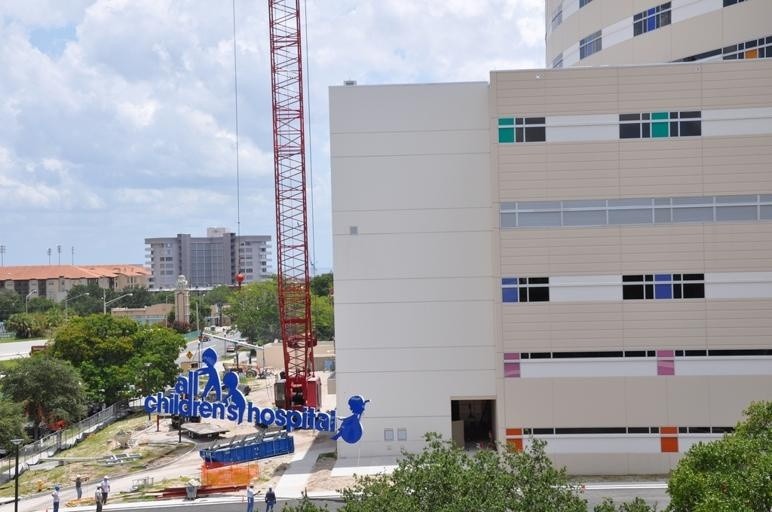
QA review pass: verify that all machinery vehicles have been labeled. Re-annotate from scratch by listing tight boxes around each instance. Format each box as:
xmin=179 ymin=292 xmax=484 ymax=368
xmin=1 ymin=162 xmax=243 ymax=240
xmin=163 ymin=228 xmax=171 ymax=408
xmin=255 ymin=0 xmax=327 ymax=437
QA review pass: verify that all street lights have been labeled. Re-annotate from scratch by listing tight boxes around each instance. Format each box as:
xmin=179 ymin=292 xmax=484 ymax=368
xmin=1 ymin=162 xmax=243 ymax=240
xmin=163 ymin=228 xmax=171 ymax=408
xmin=63 ymin=289 xmax=90 ymax=322
xmin=11 ymin=436 xmax=25 ymax=512
xmin=44 ymin=243 xmax=76 ymax=266
xmin=103 ymin=289 xmax=134 ymax=319
xmin=24 ymin=288 xmax=37 ymax=317
xmin=0 ymin=244 xmax=8 ymax=268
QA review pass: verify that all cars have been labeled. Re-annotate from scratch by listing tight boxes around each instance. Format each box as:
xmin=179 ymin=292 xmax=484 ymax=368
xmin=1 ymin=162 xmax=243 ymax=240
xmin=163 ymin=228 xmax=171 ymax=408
xmin=1 ymin=397 xmax=113 ymax=461
xmin=199 ymin=337 xmax=211 ymax=343
xmin=227 ymin=344 xmax=236 ymax=353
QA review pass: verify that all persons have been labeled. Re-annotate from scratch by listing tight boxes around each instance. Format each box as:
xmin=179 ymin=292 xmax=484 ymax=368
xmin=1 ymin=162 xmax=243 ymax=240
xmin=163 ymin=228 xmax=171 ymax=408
xmin=95 ymin=483 xmax=104 ymax=512
xmin=101 ymin=475 xmax=110 ymax=505
xmin=75 ymin=474 xmax=90 ymax=500
xmin=265 ymin=487 xmax=276 ymax=512
xmin=51 ymin=486 xmax=60 ymax=512
xmin=246 ymin=482 xmax=261 ymax=512
